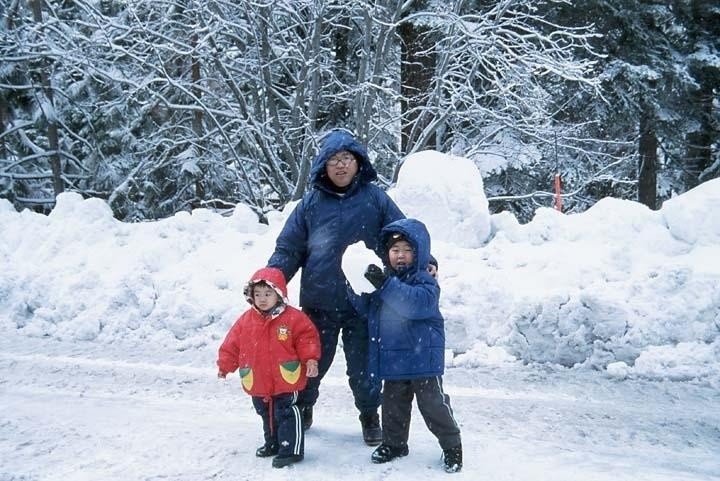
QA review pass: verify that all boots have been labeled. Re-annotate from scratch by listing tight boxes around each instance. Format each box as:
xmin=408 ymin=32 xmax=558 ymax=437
xmin=303 ymin=405 xmax=312 ymax=429
xmin=360 ymin=414 xmax=382 ymax=445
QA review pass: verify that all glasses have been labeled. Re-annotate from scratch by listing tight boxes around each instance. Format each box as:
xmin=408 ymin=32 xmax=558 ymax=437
xmin=326 ymin=155 xmax=356 ymax=167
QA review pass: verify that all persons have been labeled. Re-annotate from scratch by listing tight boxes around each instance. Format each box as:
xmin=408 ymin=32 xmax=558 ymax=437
xmin=360 ymin=217 xmax=463 ymax=474
xmin=266 ymin=130 xmax=438 ymax=446
xmin=215 ymin=266 xmax=322 ymax=467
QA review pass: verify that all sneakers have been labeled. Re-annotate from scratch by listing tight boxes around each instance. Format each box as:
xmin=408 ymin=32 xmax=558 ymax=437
xmin=273 ymin=455 xmax=304 ymax=469
xmin=371 ymin=444 xmax=409 ymax=463
xmin=256 ymin=446 xmax=279 ymax=457
xmin=442 ymin=443 xmax=462 ymax=473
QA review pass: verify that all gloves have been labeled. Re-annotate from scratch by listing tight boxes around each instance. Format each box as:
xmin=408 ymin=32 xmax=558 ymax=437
xmin=364 ymin=264 xmax=387 ymax=289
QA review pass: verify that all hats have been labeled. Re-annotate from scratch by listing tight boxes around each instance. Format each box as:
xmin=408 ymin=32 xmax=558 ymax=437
xmin=387 ymin=231 xmax=410 ymax=251
